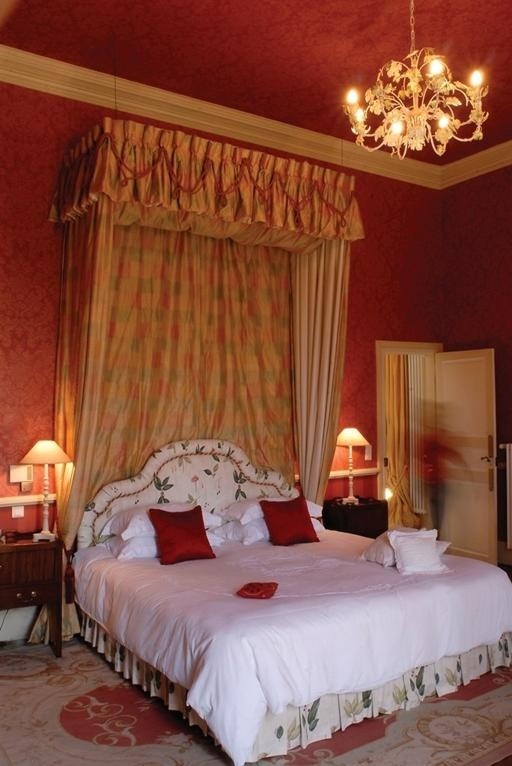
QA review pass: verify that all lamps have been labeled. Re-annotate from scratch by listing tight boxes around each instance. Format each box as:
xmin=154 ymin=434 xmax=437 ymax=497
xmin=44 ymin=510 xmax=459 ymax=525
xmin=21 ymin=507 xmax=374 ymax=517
xmin=19 ymin=439 xmax=72 ymax=544
xmin=341 ymin=0 xmax=492 ymax=161
xmin=336 ymin=427 xmax=371 ymax=506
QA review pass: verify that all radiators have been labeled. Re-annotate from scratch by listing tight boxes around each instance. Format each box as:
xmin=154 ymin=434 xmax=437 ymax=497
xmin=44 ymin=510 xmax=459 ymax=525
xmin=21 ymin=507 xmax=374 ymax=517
xmin=408 ymin=354 xmax=426 ymax=515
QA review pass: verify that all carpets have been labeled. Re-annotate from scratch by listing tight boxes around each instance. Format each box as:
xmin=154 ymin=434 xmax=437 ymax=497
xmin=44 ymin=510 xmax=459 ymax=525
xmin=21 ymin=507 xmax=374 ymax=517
xmin=0 ymin=635 xmax=512 ymax=766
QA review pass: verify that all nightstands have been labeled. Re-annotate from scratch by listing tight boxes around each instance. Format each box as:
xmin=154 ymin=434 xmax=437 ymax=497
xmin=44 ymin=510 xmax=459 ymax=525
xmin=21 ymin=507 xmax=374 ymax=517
xmin=0 ymin=538 xmax=65 ymax=657
xmin=322 ymin=495 xmax=388 ymax=540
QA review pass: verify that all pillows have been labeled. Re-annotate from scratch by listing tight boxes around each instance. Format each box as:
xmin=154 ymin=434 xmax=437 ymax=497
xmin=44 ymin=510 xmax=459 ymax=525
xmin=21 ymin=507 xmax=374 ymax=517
xmin=355 ymin=520 xmax=455 ymax=576
xmin=107 ymin=491 xmax=326 ymax=565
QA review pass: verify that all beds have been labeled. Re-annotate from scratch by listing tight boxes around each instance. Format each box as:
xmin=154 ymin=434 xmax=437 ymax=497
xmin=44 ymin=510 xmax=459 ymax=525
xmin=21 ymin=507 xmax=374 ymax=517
xmin=75 ymin=437 xmax=512 ymax=765
